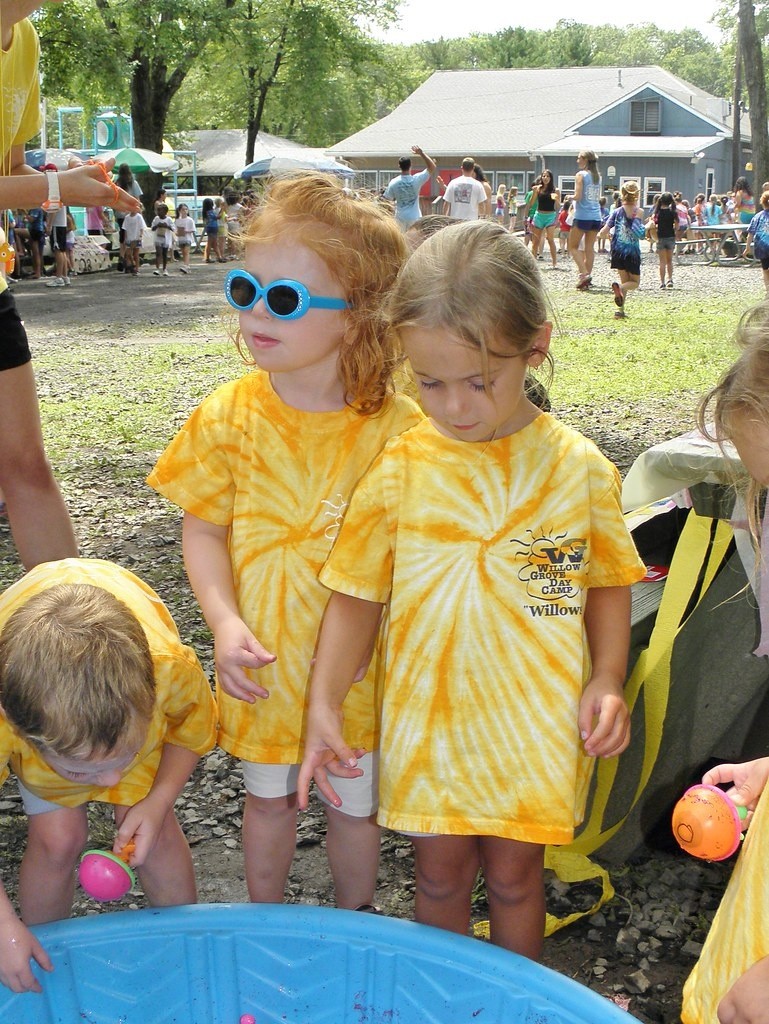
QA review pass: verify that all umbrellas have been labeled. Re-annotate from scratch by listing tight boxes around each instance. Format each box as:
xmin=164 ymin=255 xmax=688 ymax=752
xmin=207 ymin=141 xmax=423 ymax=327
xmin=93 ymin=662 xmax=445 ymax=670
xmin=25 ymin=142 xmax=183 ymax=231
xmin=232 ymin=157 xmax=355 ymax=181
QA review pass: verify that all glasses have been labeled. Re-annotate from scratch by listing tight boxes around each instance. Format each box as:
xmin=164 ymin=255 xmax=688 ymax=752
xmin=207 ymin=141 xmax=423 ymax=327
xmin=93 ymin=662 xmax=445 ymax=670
xmin=224 ymin=269 xmax=354 ymax=319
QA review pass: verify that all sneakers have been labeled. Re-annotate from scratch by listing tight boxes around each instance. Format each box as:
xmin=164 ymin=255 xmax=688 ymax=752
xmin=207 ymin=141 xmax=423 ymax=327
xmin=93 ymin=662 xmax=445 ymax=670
xmin=46 ymin=276 xmax=70 ymax=287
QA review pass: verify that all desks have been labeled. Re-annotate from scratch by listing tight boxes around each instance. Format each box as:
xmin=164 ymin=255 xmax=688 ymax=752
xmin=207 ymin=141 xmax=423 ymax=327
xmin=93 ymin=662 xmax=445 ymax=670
xmin=689 ymin=223 xmax=752 ymax=266
xmin=191 ymin=223 xmax=206 ymax=254
xmin=567 ymin=417 xmax=769 ymax=869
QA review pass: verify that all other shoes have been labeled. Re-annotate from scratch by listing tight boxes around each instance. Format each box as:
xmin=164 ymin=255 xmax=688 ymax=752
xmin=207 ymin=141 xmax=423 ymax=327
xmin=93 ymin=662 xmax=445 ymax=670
xmin=660 ymin=280 xmax=673 ymax=290
xmin=132 ymin=270 xmax=140 ymax=277
xmin=153 ymin=269 xmax=160 ymax=275
xmin=170 ymin=258 xmax=179 ymax=263
xmin=26 ymin=275 xmax=39 ymax=279
xmin=163 ymin=269 xmax=168 ymax=276
xmin=6 ymin=275 xmax=18 ymax=284
xmin=216 ymin=257 xmax=228 ymax=263
xmin=576 ymin=273 xmax=592 ymax=291
xmin=117 ymin=256 xmax=124 ymax=272
xmin=206 ymin=259 xmax=215 ymax=263
xmin=612 ymin=282 xmax=624 ymax=307
xmin=598 ymin=249 xmax=610 ymax=254
xmin=614 ymin=311 xmax=628 ymax=320
xmin=180 ymin=265 xmax=191 ymax=274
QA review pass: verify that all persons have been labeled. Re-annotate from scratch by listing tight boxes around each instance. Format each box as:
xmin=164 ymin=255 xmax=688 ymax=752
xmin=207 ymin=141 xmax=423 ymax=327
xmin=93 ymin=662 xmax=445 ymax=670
xmin=0 ymin=557 xmax=219 ymax=994
xmin=146 ymin=172 xmax=427 ymax=914
xmin=0 ymin=0 xmax=146 ymax=574
xmin=5 ymin=164 xmax=266 ymax=288
xmin=672 ymin=305 xmax=769 ymax=1024
xmin=370 ymin=145 xmax=757 ymax=269
xmin=743 ymin=182 xmax=769 ymax=308
xmin=297 ymin=219 xmax=647 ymax=965
xmin=653 ymin=192 xmax=680 ymax=290
xmin=598 ymin=181 xmax=655 ymax=320
xmin=564 ymin=150 xmax=603 ymax=291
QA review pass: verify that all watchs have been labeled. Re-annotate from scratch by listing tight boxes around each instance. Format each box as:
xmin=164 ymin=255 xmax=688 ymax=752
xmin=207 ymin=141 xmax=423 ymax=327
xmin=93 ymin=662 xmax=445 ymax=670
xmin=39 ymin=169 xmax=63 ymax=215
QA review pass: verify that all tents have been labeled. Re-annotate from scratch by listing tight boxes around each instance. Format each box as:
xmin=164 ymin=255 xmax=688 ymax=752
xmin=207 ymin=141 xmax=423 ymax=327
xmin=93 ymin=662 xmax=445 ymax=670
xmin=131 ymin=130 xmax=325 ymax=243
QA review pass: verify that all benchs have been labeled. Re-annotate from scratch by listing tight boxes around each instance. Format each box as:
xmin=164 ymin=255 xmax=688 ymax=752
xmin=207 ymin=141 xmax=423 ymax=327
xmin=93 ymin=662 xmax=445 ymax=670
xmin=627 ymin=526 xmax=736 ymax=654
xmin=675 ymin=237 xmax=763 ymax=267
